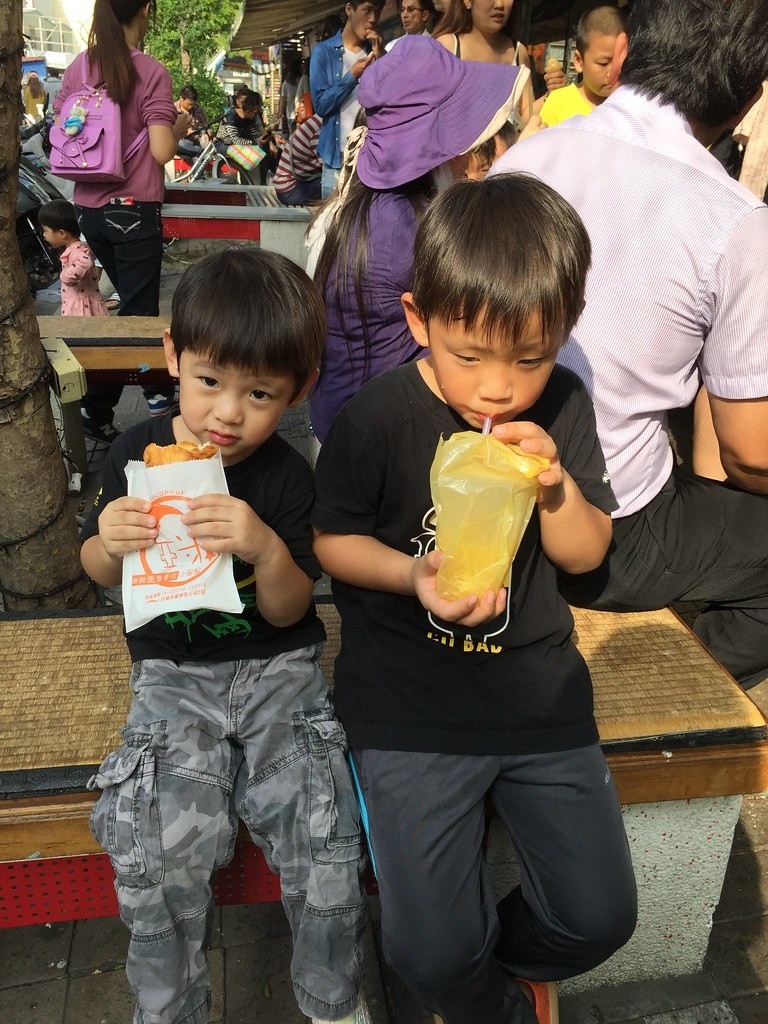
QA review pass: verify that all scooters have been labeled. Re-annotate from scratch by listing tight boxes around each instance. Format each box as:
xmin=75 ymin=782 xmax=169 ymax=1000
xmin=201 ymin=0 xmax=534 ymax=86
xmin=14 ymin=94 xmax=68 ymax=301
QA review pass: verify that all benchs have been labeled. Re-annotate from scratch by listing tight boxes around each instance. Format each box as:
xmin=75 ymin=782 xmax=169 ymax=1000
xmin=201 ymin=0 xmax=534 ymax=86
xmin=161 ymin=183 xmax=313 ymax=271
xmin=0 ymin=319 xmax=767 ymax=1004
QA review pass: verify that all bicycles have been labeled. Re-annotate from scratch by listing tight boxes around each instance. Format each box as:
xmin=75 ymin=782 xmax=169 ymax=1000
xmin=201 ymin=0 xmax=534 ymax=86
xmin=160 ymin=110 xmax=254 ymax=248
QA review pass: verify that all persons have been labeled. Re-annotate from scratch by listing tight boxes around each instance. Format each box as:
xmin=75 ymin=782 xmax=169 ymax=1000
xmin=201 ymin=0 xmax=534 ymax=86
xmin=37 ymin=199 xmax=109 ymax=316
xmin=173 ymin=85 xmax=217 ymax=180
xmin=213 ymin=84 xmax=283 ymax=185
xmin=52 ymin=0 xmax=192 ymax=447
xmin=311 ymin=170 xmax=639 ymax=1024
xmin=78 ymin=248 xmax=373 ymax=1024
xmin=271 ymin=113 xmax=324 ymax=205
xmin=539 ymin=4 xmax=623 ymax=128
xmin=309 ymin=35 xmax=549 ymax=445
xmin=21 ymin=68 xmax=62 ymax=126
xmin=483 ymin=0 xmax=768 ymax=692
xmin=277 ymin=0 xmax=567 ymax=281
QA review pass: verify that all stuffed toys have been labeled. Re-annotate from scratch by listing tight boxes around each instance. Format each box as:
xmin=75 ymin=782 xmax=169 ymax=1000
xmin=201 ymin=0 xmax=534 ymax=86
xmin=63 ymin=107 xmax=89 ymax=135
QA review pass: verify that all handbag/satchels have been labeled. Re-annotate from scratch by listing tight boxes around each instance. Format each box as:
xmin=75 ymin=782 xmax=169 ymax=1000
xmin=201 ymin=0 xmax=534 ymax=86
xmin=294 ymin=174 xmax=323 ymax=182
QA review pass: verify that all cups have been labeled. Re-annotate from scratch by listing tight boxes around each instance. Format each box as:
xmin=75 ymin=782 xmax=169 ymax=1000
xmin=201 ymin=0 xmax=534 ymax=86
xmin=437 ymin=467 xmax=533 ymax=601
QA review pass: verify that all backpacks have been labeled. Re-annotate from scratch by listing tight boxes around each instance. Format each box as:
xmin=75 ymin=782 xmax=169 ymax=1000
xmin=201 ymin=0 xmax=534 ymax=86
xmin=48 ymin=49 xmax=148 ymax=180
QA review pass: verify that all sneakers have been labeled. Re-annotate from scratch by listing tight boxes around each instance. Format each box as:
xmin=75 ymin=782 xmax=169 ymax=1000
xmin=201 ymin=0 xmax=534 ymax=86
xmin=147 ymin=391 xmax=179 ymax=417
xmin=80 ymin=408 xmax=122 ymax=444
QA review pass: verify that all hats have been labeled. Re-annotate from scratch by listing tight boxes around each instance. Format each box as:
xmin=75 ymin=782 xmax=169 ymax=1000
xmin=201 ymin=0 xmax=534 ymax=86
xmin=357 ymin=35 xmax=530 ymax=188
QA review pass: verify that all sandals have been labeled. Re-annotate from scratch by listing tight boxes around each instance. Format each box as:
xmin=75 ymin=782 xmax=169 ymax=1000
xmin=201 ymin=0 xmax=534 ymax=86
xmin=106 ymin=293 xmax=121 ymax=309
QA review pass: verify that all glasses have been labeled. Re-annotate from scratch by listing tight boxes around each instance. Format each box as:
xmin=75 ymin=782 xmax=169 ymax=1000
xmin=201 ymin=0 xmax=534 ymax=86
xmin=399 ymin=6 xmax=424 ymax=13
